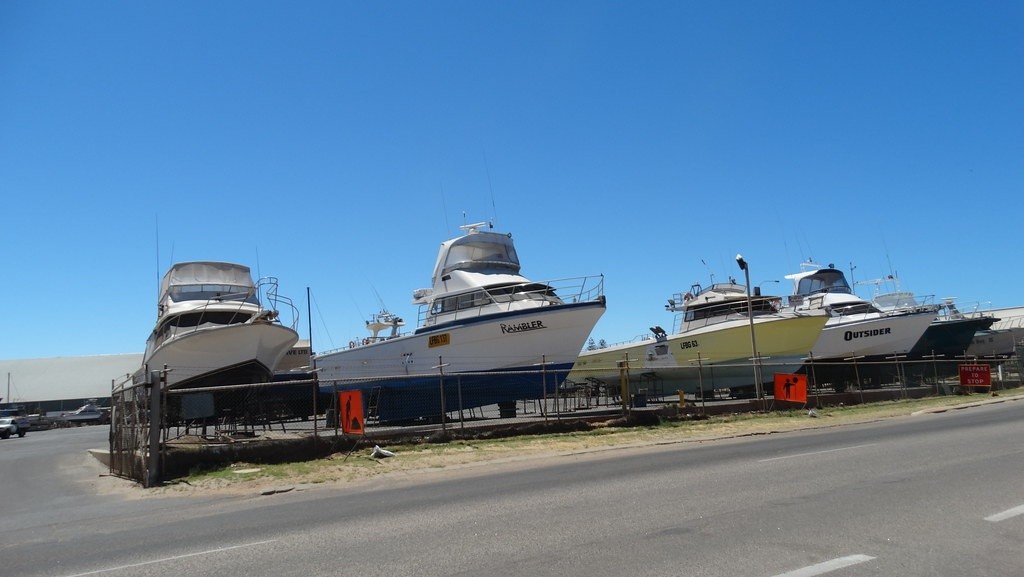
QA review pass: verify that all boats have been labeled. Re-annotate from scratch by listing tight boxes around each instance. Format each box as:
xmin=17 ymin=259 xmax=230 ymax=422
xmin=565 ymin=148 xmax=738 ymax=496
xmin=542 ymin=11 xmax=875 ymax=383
xmin=131 ymin=261 xmax=301 ymax=422
xmin=315 ymin=212 xmax=608 ymax=421
xmin=910 ymin=293 xmax=1023 ymax=380
xmin=784 ymin=255 xmax=947 ymax=378
xmin=560 ymin=260 xmax=833 ymax=400
xmin=51 ymin=398 xmax=112 ymax=423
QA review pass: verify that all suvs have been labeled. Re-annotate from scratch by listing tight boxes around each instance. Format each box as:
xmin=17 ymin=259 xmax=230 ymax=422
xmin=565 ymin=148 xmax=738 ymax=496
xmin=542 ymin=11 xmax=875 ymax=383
xmin=0 ymin=409 xmax=31 ymax=440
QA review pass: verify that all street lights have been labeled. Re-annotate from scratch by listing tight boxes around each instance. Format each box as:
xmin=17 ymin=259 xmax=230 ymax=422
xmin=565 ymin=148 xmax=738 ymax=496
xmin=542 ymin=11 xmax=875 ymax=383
xmin=735 ymin=254 xmax=762 ymax=402
